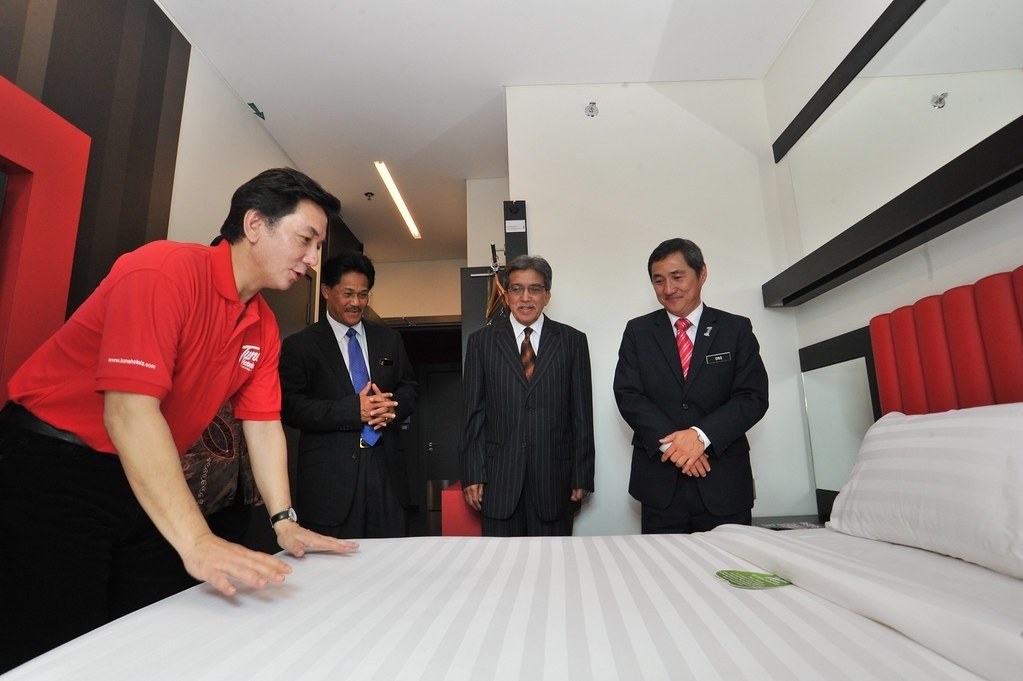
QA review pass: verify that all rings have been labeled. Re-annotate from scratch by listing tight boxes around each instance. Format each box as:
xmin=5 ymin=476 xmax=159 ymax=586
xmin=386 ymin=418 xmax=387 ymax=421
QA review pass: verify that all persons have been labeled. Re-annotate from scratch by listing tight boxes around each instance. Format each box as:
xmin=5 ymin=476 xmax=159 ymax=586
xmin=0 ymin=168 xmax=360 ymax=673
xmin=458 ymin=256 xmax=595 ymax=536
xmin=277 ymin=250 xmax=420 ymax=540
xmin=613 ymin=238 xmax=769 ymax=534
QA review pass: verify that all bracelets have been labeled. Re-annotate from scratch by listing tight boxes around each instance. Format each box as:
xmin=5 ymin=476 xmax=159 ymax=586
xmin=697 ymin=431 xmax=704 ymax=443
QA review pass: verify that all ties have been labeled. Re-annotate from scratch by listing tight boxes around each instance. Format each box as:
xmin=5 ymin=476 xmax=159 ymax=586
xmin=520 ymin=328 xmax=536 ymax=381
xmin=346 ymin=328 xmax=383 ymax=447
xmin=675 ymin=318 xmax=693 ymax=382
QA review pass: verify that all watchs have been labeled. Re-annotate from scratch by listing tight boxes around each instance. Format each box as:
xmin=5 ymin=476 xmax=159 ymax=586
xmin=270 ymin=508 xmax=297 ymax=526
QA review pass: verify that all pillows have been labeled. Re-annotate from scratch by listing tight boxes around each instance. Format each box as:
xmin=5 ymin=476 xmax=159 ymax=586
xmin=825 ymin=401 xmax=1023 ymax=580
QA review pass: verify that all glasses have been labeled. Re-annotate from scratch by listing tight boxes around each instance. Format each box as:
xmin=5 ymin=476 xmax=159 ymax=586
xmin=329 ymin=287 xmax=372 ymax=299
xmin=507 ymin=285 xmax=546 ymax=295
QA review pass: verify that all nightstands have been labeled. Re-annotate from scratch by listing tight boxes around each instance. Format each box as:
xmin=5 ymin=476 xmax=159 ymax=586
xmin=751 ymin=515 xmax=830 ymax=532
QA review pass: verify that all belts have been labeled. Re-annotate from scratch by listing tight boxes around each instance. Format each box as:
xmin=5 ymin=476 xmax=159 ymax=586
xmin=0 ymin=399 xmax=92 ymax=450
xmin=359 ymin=437 xmax=382 ymax=448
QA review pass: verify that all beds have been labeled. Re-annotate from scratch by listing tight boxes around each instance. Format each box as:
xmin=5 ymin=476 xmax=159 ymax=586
xmin=0 ymin=524 xmax=1023 ymax=681
